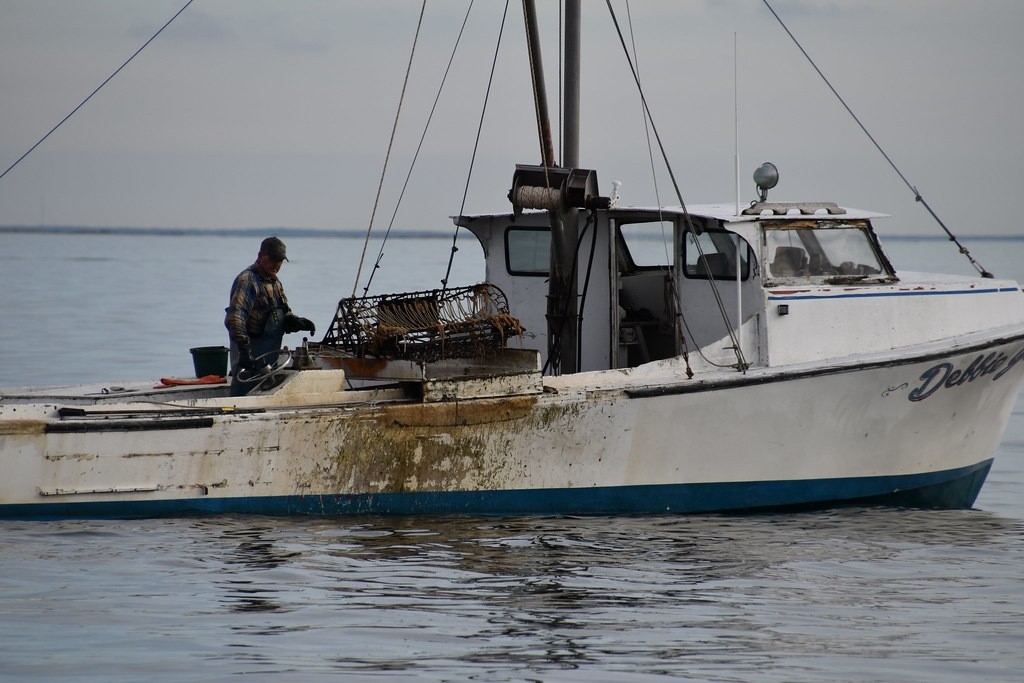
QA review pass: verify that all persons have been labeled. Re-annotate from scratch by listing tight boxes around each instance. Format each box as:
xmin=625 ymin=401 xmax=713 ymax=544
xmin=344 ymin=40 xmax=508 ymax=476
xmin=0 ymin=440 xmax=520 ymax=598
xmin=223 ymin=235 xmax=315 ymax=396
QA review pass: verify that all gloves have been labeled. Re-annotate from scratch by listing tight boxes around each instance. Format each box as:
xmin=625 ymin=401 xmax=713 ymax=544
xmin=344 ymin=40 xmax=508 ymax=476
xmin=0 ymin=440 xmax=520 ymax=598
xmin=300 ymin=317 xmax=316 ymax=337
xmin=238 ymin=345 xmax=254 ymax=369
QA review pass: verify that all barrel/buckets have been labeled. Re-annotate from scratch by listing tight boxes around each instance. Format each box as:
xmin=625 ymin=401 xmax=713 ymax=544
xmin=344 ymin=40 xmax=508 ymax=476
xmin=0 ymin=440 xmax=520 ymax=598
xmin=189 ymin=346 xmax=230 ymax=379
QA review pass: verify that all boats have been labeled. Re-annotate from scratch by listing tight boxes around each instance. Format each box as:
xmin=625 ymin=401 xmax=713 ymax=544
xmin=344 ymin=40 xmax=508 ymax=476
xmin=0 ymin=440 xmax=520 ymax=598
xmin=1 ymin=0 xmax=1024 ymax=518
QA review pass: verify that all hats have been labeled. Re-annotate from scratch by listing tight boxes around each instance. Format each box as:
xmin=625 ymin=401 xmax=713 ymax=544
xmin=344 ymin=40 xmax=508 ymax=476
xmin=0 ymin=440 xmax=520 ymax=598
xmin=261 ymin=236 xmax=289 ymax=263
